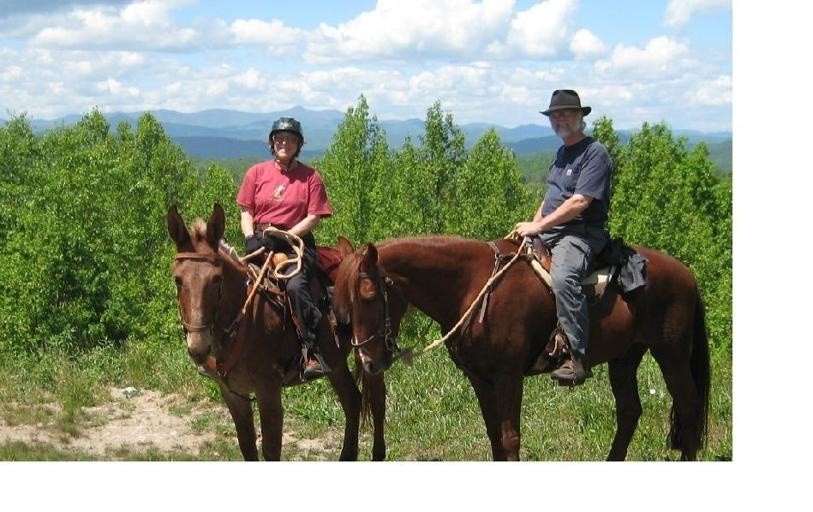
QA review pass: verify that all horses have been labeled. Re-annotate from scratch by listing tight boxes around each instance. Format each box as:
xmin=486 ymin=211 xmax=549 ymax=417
xmin=333 ymin=235 xmax=711 ymax=461
xmin=167 ymin=203 xmax=385 ymax=461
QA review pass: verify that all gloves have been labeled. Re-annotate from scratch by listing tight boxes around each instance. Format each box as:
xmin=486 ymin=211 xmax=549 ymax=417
xmin=246 ymin=235 xmax=261 ymax=262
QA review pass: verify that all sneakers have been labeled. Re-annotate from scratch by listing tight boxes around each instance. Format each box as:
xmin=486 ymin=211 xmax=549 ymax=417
xmin=304 ymin=358 xmax=332 ymax=378
xmin=552 ymin=359 xmax=594 ymax=381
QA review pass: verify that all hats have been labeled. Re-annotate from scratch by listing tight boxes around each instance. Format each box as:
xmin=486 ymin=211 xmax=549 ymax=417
xmin=539 ymin=90 xmax=592 ymax=115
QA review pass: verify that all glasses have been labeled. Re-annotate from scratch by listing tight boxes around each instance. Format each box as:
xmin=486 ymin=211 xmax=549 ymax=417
xmin=272 ymin=134 xmax=299 ymax=143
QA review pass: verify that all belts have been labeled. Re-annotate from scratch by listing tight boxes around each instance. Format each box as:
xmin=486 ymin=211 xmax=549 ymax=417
xmin=255 ymin=222 xmax=285 ymax=231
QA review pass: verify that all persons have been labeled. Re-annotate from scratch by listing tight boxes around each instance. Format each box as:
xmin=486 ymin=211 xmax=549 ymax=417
xmin=237 ymin=117 xmax=332 ymax=378
xmin=516 ymin=89 xmax=614 ymax=379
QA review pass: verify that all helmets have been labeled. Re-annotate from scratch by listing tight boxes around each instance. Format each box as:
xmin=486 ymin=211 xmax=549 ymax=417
xmin=269 ymin=117 xmax=304 ymax=148
